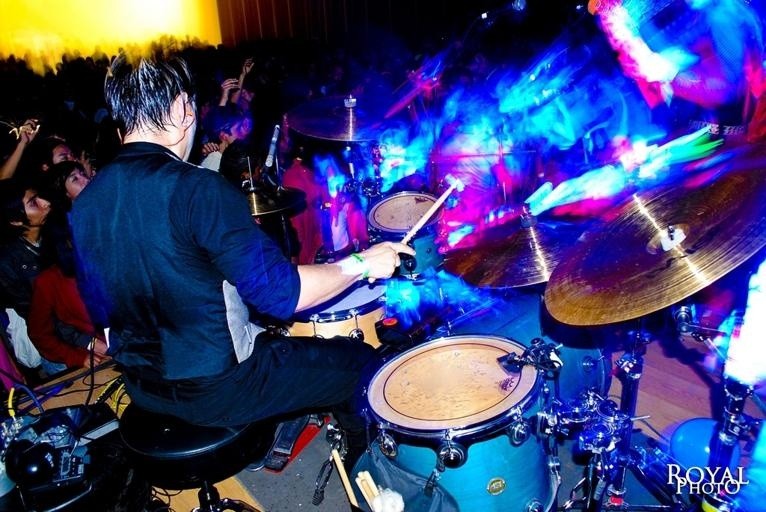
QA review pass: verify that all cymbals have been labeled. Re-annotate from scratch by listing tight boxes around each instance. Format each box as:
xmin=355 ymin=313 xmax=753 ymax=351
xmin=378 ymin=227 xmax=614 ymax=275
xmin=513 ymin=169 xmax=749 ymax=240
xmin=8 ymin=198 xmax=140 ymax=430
xmin=285 ymin=92 xmax=408 ymax=145
xmin=238 ymin=186 xmax=307 ymax=216
xmin=442 ymin=167 xmax=762 ymax=325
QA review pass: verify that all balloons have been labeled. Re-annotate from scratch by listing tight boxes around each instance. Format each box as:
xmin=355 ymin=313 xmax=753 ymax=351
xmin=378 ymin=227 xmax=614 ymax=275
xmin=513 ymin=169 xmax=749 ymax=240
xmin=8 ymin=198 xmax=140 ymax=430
xmin=671 ymin=418 xmax=743 ymax=481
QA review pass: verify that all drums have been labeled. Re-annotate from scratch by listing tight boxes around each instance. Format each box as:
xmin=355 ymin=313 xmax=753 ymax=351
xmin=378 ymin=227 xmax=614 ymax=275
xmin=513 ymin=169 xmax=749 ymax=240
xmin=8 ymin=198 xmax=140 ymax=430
xmin=424 ymin=284 xmax=613 ymax=434
xmin=267 ymin=282 xmax=388 ymax=349
xmin=368 ymin=190 xmax=449 ymax=284
xmin=358 ymin=335 xmax=562 ymax=512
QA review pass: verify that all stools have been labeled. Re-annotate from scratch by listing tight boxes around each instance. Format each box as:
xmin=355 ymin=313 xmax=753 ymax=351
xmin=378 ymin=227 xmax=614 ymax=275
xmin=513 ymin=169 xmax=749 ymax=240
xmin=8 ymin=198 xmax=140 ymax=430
xmin=120 ymin=401 xmax=276 ymax=512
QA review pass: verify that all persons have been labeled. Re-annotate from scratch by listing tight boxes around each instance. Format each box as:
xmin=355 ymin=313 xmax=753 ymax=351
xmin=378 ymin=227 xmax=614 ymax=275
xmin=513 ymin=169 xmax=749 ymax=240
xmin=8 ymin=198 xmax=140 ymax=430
xmin=0 ymin=57 xmax=342 ymax=391
xmin=69 ymin=48 xmax=415 ymax=510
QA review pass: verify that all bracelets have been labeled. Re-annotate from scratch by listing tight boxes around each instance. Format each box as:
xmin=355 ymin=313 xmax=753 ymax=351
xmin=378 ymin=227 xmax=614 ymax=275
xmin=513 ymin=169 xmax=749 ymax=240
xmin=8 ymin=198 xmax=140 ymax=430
xmin=350 ymin=252 xmax=370 ymax=282
